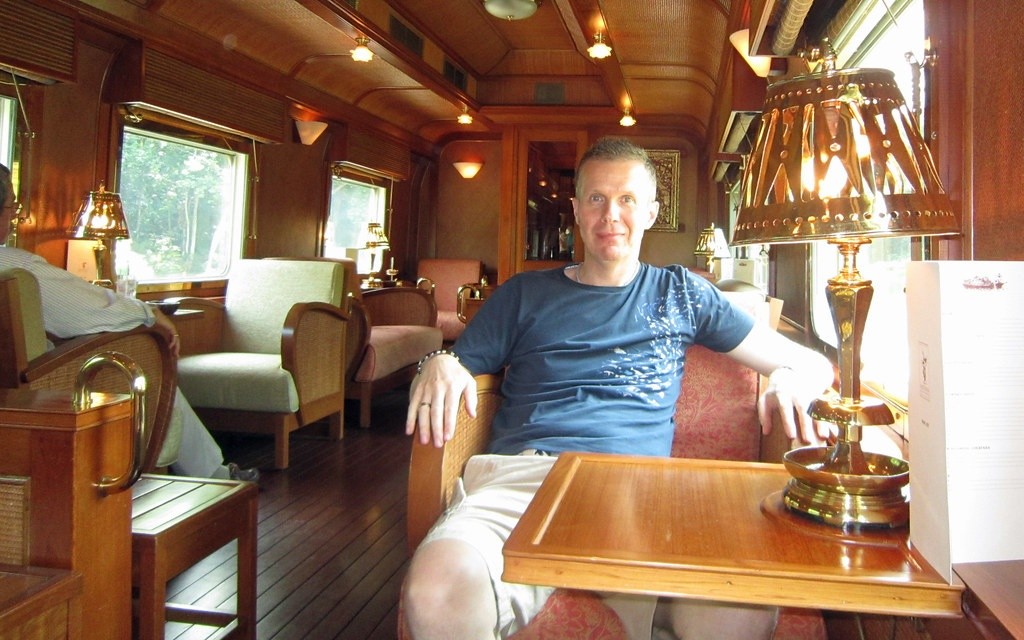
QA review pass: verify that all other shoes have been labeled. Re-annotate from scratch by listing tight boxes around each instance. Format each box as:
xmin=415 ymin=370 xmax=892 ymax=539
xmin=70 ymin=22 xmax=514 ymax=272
xmin=227 ymin=463 xmax=259 ymax=481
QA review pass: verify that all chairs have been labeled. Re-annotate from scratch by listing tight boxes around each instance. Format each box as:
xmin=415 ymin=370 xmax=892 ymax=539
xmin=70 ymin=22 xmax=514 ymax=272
xmin=0 ymin=259 xmax=862 ymax=640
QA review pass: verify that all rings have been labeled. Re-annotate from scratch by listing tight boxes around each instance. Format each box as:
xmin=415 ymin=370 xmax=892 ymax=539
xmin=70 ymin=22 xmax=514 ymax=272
xmin=174 ymin=333 xmax=179 ymax=336
xmin=419 ymin=402 xmax=431 ymax=409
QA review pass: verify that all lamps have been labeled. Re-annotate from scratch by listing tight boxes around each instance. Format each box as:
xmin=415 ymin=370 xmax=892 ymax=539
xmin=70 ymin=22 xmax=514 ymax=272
xmin=692 ymin=222 xmax=730 ymax=275
xmin=729 ymin=28 xmax=771 ymax=77
xmin=351 ymin=37 xmax=373 ymax=62
xmin=484 ymin=0 xmax=537 ymax=22
xmin=586 ymin=32 xmax=612 ymax=59
xmin=728 ymin=37 xmax=961 ymax=522
xmin=620 ymin=109 xmax=636 ymax=126
xmin=65 ymin=183 xmax=130 ymax=288
xmin=295 ymin=121 xmax=328 ymax=145
xmin=457 ymin=107 xmax=473 ymax=125
xmin=361 ymin=222 xmax=391 ymax=290
xmin=453 ymin=162 xmax=482 ymax=179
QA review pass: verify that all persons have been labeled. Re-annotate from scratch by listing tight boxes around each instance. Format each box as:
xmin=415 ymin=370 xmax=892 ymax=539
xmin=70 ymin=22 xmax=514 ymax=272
xmin=405 ymin=139 xmax=841 ymax=640
xmin=0 ymin=163 xmax=260 ymax=483
xmin=545 ymin=206 xmax=559 ymax=260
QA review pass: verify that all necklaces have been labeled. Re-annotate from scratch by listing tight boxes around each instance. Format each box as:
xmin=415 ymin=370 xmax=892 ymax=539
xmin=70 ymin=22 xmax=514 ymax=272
xmin=577 ymin=261 xmax=584 ymax=285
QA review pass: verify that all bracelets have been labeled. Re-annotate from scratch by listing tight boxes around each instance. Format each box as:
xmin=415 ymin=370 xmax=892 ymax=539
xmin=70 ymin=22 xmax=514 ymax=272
xmin=782 ymin=366 xmax=793 ymax=371
xmin=417 ymin=349 xmax=460 ymax=376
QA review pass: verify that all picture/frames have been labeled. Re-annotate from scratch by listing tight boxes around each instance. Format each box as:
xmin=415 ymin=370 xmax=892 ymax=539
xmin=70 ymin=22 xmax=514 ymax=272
xmin=644 ymin=150 xmax=680 ymax=233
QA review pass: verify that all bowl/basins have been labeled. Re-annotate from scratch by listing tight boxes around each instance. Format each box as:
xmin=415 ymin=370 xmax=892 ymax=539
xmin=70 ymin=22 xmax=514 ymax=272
xmin=146 ymin=300 xmax=180 ymax=315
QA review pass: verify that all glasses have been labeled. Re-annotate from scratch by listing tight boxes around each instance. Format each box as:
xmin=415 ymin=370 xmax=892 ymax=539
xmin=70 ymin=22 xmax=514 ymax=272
xmin=0 ymin=202 xmax=23 ymax=214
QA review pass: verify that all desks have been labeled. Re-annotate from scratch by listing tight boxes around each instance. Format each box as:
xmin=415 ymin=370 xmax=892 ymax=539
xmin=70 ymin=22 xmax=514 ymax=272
xmin=501 ymin=451 xmax=966 ymax=640
xmin=131 ymin=474 xmax=259 ymax=640
xmin=145 ymin=308 xmax=206 ymax=322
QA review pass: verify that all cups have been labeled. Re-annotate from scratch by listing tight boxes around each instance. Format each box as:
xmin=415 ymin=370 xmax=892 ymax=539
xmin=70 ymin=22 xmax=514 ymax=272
xmin=116 ymin=266 xmax=136 ymax=299
